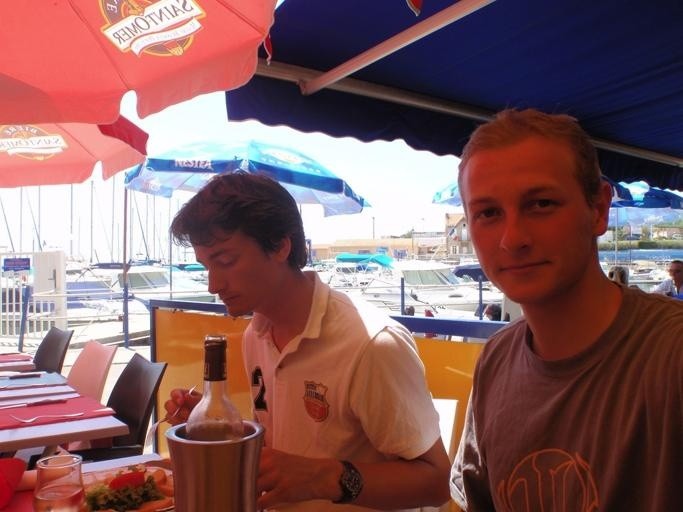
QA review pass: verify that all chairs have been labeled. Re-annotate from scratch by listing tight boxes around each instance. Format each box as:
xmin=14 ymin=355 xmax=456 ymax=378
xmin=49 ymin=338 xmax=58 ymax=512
xmin=67 ymin=343 xmax=116 ymax=450
xmin=28 ymin=354 xmax=168 ymax=470
xmin=32 ymin=325 xmax=74 ymax=374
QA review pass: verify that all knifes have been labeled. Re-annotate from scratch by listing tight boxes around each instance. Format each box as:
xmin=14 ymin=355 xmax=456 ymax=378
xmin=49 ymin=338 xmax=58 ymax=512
xmin=0 ymin=373 xmax=41 ymax=380
xmin=0 ymin=399 xmax=67 ymax=410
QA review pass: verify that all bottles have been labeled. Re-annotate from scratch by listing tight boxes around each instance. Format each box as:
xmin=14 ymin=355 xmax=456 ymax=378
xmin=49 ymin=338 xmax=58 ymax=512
xmin=182 ymin=332 xmax=245 ymax=443
xmin=33 ymin=452 xmax=87 ymax=511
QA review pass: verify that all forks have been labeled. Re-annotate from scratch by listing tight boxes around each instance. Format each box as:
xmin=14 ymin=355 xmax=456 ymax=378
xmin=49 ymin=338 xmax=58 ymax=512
xmin=8 ymin=412 xmax=84 ymax=423
xmin=139 ymin=383 xmax=197 ymax=445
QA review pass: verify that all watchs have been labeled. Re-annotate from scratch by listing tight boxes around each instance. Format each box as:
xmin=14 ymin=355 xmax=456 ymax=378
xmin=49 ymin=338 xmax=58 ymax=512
xmin=333 ymin=460 xmax=363 ymax=503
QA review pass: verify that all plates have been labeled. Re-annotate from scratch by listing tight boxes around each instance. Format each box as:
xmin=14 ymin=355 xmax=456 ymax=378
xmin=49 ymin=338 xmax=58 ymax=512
xmin=79 ymin=464 xmax=175 ymax=512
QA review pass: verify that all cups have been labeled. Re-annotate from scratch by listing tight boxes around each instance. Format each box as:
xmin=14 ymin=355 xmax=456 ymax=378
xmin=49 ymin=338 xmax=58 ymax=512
xmin=163 ymin=417 xmax=266 ymax=512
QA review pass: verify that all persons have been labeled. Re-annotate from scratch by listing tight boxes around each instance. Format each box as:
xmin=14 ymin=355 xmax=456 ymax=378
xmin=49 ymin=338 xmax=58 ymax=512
xmin=165 ymin=172 xmax=450 ymax=512
xmin=449 ymin=109 xmax=683 ymax=512
xmin=651 ymin=261 xmax=683 ymax=300
xmin=608 ymin=265 xmax=627 ymax=286
